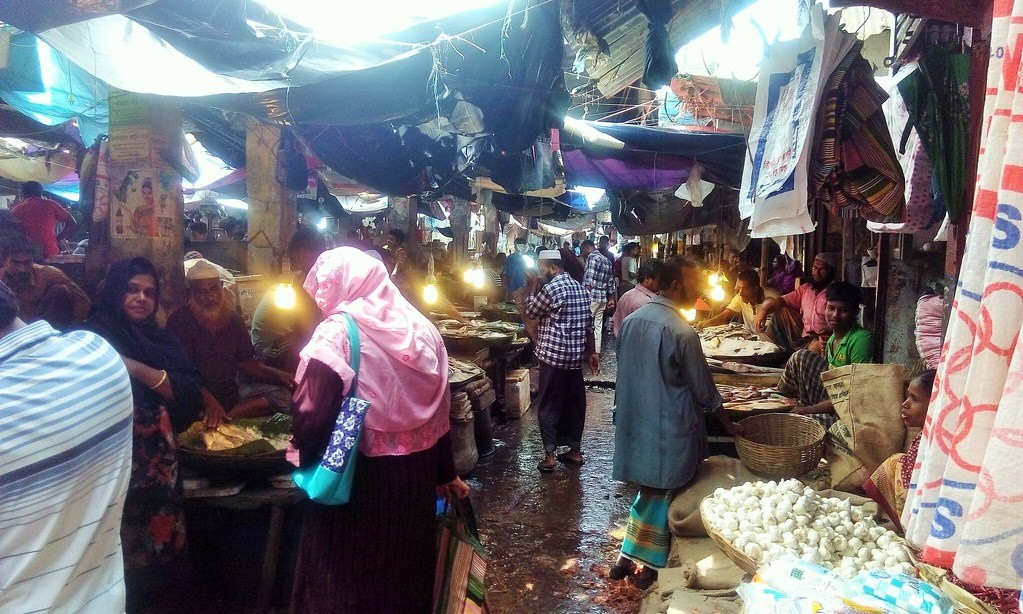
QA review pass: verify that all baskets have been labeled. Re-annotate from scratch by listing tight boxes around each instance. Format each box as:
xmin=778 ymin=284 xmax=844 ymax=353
xmin=175 ymin=448 xmax=287 ymax=474
xmin=736 ymin=412 xmax=826 ymax=479
xmin=701 ymin=492 xmax=760 ymax=578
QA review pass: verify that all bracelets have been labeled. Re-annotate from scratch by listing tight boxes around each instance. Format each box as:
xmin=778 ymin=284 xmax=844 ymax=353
xmin=150 ymin=370 xmax=167 ymax=390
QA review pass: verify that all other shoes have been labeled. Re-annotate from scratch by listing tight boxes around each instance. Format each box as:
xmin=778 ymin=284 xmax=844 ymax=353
xmin=636 ymin=569 xmax=658 ymax=589
xmin=609 ymin=562 xmax=635 ymax=580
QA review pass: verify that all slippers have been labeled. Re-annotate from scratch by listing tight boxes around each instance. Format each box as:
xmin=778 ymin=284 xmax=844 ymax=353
xmin=557 ymin=452 xmax=583 ymax=464
xmin=538 ymin=461 xmax=554 ymax=471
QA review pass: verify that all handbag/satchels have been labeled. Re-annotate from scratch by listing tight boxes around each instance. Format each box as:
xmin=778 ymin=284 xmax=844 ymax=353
xmin=290 ymin=311 xmax=371 ymax=506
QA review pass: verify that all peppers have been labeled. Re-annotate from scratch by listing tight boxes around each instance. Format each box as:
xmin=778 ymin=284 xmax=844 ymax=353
xmin=945 ymin=567 xmax=1022 ymax=614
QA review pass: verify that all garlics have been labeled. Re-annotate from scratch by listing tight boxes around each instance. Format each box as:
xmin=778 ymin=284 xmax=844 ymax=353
xmin=701 ymin=477 xmax=917 ymax=580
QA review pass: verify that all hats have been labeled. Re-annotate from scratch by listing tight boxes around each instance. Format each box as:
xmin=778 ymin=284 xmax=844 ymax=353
xmin=186 ymin=261 xmax=220 ymax=279
xmin=538 ymin=250 xmax=562 ymax=260
xmin=815 ymin=252 xmax=836 ymax=267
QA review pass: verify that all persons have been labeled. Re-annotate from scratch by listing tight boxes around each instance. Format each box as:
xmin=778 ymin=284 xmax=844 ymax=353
xmin=365 ymin=228 xmax=470 ymax=326
xmin=90 ymin=255 xmax=206 ymax=614
xmin=220 ymin=218 xmax=238 ymax=240
xmin=613 ymin=258 xmax=663 ymax=423
xmin=681 ymin=243 xmax=873 ymax=432
xmin=608 ymin=257 xmax=744 ymax=588
xmin=0 ymin=208 xmax=134 ymax=614
xmin=184 ymin=251 xmax=237 ymax=313
xmin=464 ymin=262 xmax=503 ymax=301
xmin=165 ymin=262 xmax=297 ymax=428
xmin=250 ymin=263 xmax=319 ymax=370
xmin=119 ymin=177 xmax=157 ymax=238
xmin=864 ymin=369 xmax=937 ymax=532
xmin=288 ymin=245 xmax=470 ymax=614
xmin=11 ymin=180 xmax=77 ymax=257
xmin=523 ymin=250 xmax=601 ymax=471
xmin=497 ymin=236 xmax=641 ymax=344
xmin=189 ymin=222 xmax=207 ymax=240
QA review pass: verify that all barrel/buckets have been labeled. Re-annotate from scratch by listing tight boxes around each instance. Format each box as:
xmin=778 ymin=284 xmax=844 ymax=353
xmin=449 ymin=418 xmax=478 ymax=475
xmin=473 ymin=410 xmax=495 ymax=457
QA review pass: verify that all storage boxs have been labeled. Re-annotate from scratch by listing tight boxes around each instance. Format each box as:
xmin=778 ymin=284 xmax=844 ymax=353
xmin=505 ymin=369 xmax=531 ymax=416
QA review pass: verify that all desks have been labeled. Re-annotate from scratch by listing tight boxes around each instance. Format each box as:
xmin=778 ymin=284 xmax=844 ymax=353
xmin=178 ymin=482 xmax=304 ymax=614
